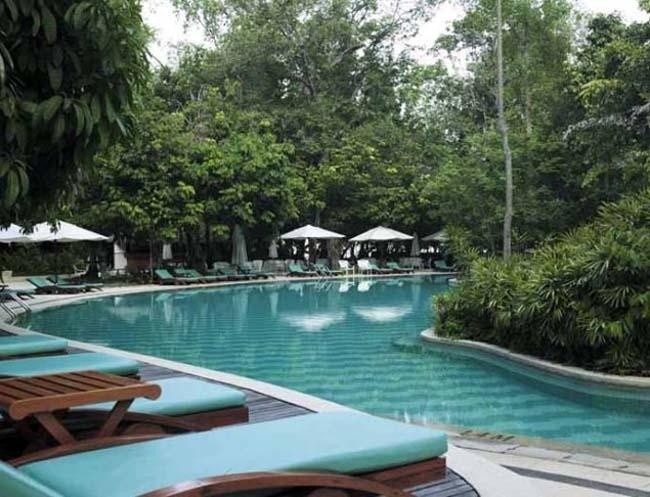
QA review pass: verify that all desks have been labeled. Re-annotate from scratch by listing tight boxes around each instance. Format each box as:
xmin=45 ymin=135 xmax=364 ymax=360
xmin=1 ymin=370 xmax=214 ymax=467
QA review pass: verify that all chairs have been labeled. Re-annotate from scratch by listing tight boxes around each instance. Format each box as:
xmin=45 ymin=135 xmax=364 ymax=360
xmin=0 ymin=376 xmax=250 ymax=460
xmin=0 ymin=407 xmax=449 ymax=495
xmin=0 ymin=350 xmax=144 ymax=380
xmin=0 ymin=330 xmax=70 ymax=360
xmin=0 ymin=256 xmax=473 ymax=302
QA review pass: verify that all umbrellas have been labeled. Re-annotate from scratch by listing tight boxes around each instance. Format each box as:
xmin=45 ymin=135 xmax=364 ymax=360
xmin=268 ymin=239 xmax=279 ymax=259
xmin=0 ymin=222 xmax=41 ymax=245
xmin=277 ymin=293 xmax=349 ymax=335
xmin=348 ymin=300 xmax=415 ymax=325
xmin=347 ymin=225 xmax=416 ymax=265
xmin=278 ymin=223 xmax=347 ymax=263
xmin=231 ymin=291 xmax=250 ymax=322
xmin=410 ymin=281 xmax=422 ymax=308
xmin=162 ymin=291 xmax=175 ymax=324
xmin=161 ymin=242 xmax=173 ymax=261
xmin=103 ymin=296 xmax=146 ymax=325
xmin=230 ymin=223 xmax=249 ymax=274
xmin=28 ymin=217 xmax=112 ymax=283
xmin=421 ymin=228 xmax=450 ymax=241
xmin=269 ymin=291 xmax=279 ymax=320
